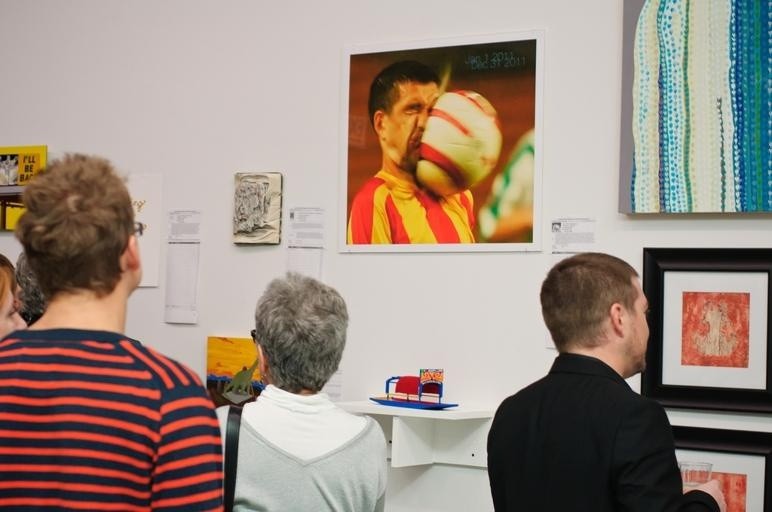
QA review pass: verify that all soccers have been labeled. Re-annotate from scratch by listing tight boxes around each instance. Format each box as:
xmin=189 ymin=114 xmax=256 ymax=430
xmin=416 ymin=92 xmax=501 ymax=197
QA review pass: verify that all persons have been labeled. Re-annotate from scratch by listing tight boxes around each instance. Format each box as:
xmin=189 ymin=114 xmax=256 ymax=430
xmin=346 ymin=60 xmax=476 ymax=243
xmin=478 ymin=128 xmax=534 ymax=242
xmin=0 ymin=153 xmax=224 ymax=512
xmin=15 ymin=249 xmax=48 ymax=326
xmin=215 ymin=272 xmax=388 ymax=512
xmin=486 ymin=253 xmax=728 ymax=512
xmin=0 ymin=254 xmax=27 ymax=340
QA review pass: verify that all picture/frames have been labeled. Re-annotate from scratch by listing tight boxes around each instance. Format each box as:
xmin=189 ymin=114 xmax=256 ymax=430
xmin=671 ymin=426 xmax=772 ymax=512
xmin=641 ymin=248 xmax=772 ymax=413
